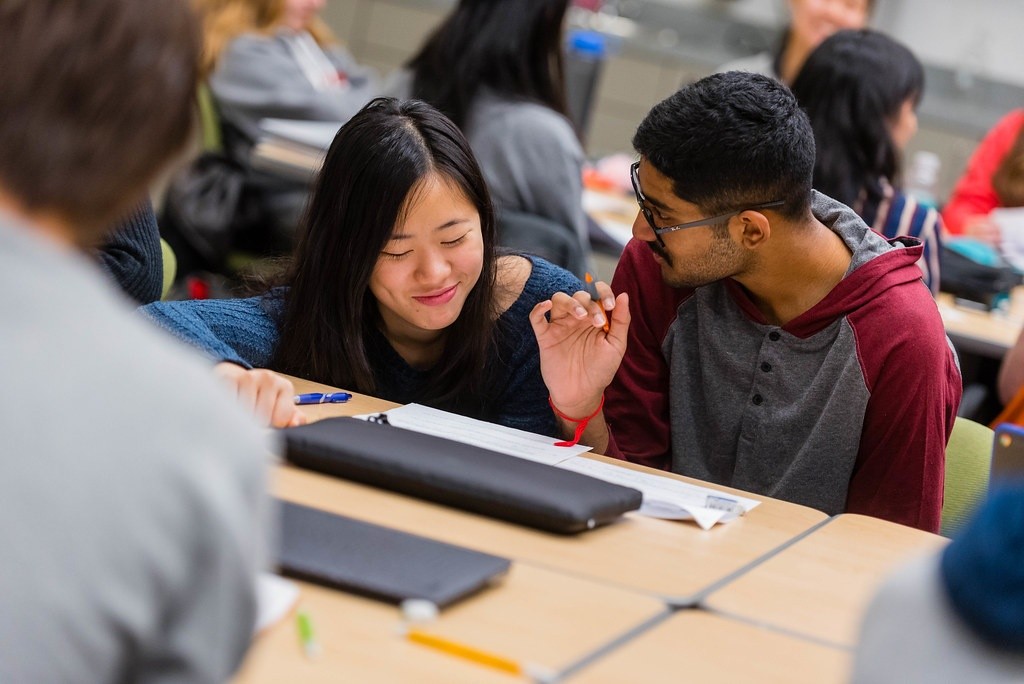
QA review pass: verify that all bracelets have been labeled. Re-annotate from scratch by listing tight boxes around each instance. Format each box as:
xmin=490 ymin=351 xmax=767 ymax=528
xmin=548 ymin=392 xmax=604 ymax=447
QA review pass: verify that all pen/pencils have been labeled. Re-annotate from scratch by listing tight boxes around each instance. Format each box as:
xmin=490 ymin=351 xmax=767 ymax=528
xmin=392 ymin=626 xmax=557 ymax=682
xmin=585 ymin=272 xmax=609 ymax=332
xmin=297 ymin=610 xmax=317 ymax=661
xmin=293 ymin=391 xmax=353 ymax=405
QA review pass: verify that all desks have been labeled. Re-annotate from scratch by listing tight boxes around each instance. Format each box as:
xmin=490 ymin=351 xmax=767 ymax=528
xmin=256 ymin=369 xmax=832 ymax=606
xmin=697 ymin=511 xmax=953 ymax=649
xmin=233 ymin=561 xmax=673 ymax=682
xmin=249 ymin=131 xmax=1024 ymax=364
xmin=565 ymin=607 xmax=850 ymax=682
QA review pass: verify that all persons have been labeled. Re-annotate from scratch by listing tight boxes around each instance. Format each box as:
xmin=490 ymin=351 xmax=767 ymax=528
xmin=789 ymin=30 xmax=944 ymax=302
xmin=382 ymin=0 xmax=592 ymax=275
xmin=141 ymin=96 xmax=597 ymax=441
xmin=202 ymin=1 xmax=406 ymax=121
xmin=0 ymin=1 xmax=278 ymax=684
xmin=530 ymin=71 xmax=963 ymax=535
xmin=944 ymin=105 xmax=1024 ymax=235
xmin=698 ymin=0 xmax=874 ymax=90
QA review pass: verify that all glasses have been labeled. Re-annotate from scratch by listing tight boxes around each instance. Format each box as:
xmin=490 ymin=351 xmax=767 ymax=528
xmin=630 ymin=161 xmax=784 ymax=246
